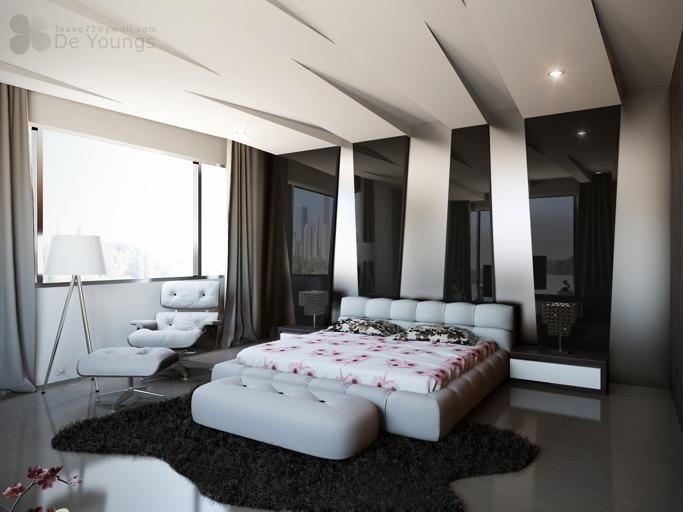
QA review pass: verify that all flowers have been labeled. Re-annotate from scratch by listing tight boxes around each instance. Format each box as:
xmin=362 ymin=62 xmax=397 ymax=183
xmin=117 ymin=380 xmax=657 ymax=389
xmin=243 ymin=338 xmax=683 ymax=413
xmin=0 ymin=466 xmax=83 ymax=512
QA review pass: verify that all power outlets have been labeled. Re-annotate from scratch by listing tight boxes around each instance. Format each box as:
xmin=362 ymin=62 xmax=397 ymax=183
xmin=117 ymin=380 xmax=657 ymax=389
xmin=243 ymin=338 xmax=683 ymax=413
xmin=56 ymin=365 xmax=65 ymax=375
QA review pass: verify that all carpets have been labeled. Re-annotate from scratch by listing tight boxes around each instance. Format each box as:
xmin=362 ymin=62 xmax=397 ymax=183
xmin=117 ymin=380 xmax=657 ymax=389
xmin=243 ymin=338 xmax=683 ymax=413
xmin=52 ymin=390 xmax=540 ymax=511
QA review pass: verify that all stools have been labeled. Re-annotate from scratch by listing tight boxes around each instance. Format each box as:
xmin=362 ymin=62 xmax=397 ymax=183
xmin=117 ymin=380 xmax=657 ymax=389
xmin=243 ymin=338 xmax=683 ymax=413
xmin=77 ymin=347 xmax=179 ymax=410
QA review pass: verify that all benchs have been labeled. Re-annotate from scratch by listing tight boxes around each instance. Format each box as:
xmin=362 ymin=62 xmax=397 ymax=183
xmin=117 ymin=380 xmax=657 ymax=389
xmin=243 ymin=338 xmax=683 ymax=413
xmin=191 ymin=376 xmax=381 ymax=461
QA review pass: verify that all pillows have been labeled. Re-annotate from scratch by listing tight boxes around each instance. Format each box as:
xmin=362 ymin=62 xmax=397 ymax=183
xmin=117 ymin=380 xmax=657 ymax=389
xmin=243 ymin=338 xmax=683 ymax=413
xmin=326 ymin=319 xmax=405 ymax=337
xmin=394 ymin=325 xmax=479 ymax=346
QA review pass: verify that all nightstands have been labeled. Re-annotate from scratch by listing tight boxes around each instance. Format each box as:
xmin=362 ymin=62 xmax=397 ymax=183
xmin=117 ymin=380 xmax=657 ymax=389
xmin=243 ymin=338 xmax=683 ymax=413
xmin=511 ymin=344 xmax=610 ymax=395
xmin=277 ymin=324 xmax=327 ymax=340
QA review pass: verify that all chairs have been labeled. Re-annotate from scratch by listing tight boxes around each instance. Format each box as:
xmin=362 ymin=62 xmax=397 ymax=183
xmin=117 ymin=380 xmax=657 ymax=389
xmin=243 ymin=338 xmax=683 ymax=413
xmin=127 ymin=280 xmax=223 ymax=381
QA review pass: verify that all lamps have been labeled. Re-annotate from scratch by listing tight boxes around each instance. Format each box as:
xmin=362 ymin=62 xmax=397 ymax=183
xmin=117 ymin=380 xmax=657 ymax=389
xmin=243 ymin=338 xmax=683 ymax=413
xmin=41 ymin=236 xmax=106 ymax=394
xmin=299 ymin=290 xmax=329 ymax=331
xmin=540 ymin=302 xmax=583 ymax=356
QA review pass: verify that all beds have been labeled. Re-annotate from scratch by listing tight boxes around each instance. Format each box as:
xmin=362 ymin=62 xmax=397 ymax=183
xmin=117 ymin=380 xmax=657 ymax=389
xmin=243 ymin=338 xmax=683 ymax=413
xmin=211 ymin=297 xmax=516 ymax=442
